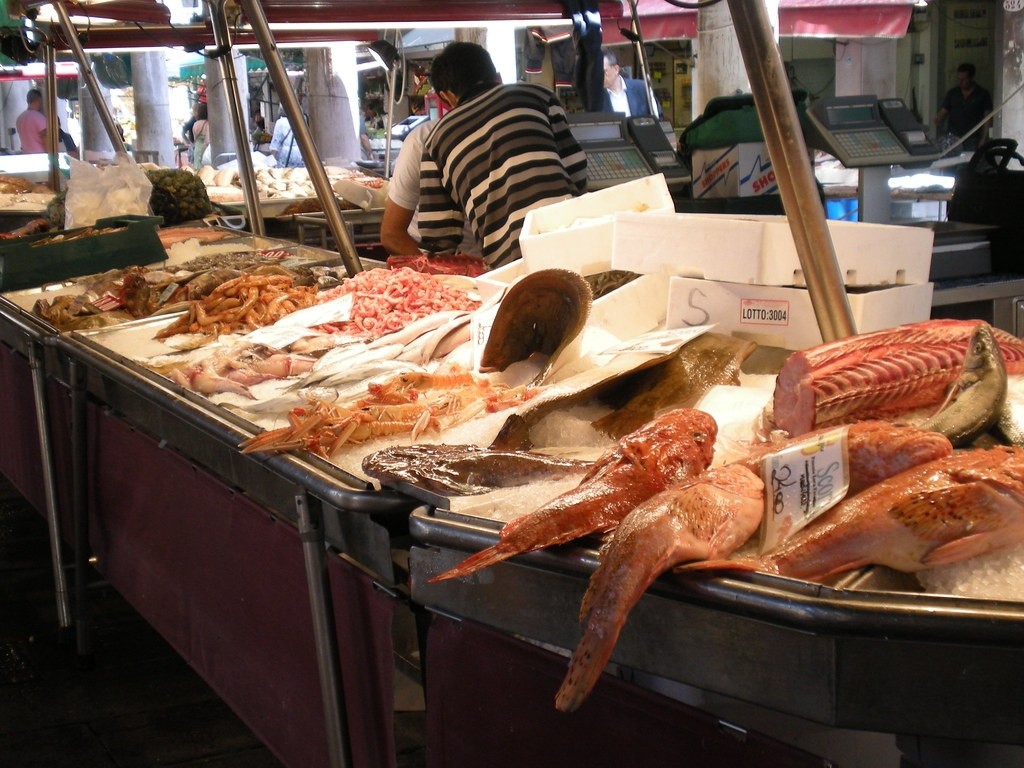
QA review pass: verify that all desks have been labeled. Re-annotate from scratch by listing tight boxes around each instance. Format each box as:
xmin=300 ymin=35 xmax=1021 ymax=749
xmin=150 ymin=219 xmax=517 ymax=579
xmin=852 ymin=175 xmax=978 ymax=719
xmin=5 ymin=224 xmax=1023 ymax=768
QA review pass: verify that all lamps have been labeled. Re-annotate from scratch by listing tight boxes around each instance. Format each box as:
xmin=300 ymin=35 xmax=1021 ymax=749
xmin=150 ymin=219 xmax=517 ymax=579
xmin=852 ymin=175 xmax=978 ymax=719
xmin=368 ymin=40 xmax=401 ymax=72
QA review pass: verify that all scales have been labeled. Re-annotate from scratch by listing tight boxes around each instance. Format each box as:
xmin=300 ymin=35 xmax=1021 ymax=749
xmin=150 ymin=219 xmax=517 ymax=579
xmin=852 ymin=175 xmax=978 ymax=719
xmin=353 ymin=114 xmax=430 ymax=178
xmin=806 ymin=93 xmax=996 ymax=282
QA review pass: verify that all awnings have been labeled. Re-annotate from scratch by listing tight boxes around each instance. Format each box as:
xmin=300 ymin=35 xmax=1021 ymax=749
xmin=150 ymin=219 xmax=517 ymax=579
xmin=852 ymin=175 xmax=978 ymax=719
xmin=602 ymin=0 xmax=928 ymax=46
xmin=179 ymin=47 xmax=304 ymax=81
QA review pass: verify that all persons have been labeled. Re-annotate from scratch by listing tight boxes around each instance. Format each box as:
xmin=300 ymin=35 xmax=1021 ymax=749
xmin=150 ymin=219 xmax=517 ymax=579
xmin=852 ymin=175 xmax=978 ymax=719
xmin=380 ymin=118 xmax=487 ymax=257
xmin=193 ymin=104 xmax=210 ymax=171
xmin=269 ymin=101 xmax=310 ymax=165
xmin=183 ymin=103 xmax=197 ymax=165
xmin=602 ymin=53 xmax=661 ymax=119
xmin=359 ymin=97 xmax=375 ymax=160
xmin=16 ymin=90 xmax=46 ymax=153
xmin=932 ymin=62 xmax=993 ymax=148
xmin=58 ymin=110 xmax=76 ymax=152
xmin=418 ymin=41 xmax=586 ymax=270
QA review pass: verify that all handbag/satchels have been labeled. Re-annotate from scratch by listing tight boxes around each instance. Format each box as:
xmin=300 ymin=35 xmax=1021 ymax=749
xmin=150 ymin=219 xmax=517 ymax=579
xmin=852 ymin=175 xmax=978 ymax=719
xmin=64 ymin=151 xmax=153 ymax=230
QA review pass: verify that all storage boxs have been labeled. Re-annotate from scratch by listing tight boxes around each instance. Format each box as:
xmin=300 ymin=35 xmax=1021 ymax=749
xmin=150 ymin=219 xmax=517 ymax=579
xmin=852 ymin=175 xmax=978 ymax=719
xmin=1 ymin=214 xmax=170 ymax=293
xmin=691 ymin=141 xmax=782 ymax=198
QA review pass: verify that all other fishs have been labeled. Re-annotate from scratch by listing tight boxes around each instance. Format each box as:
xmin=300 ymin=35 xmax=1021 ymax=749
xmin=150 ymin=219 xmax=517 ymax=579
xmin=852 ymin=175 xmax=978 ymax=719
xmin=72 ymin=262 xmax=210 ymax=318
xmin=428 ymin=314 xmax=1024 ymax=711
xmin=138 ymin=160 xmax=318 ymax=205
xmin=165 ymin=336 xmax=341 ymax=403
xmin=217 ymin=309 xmax=475 ymax=417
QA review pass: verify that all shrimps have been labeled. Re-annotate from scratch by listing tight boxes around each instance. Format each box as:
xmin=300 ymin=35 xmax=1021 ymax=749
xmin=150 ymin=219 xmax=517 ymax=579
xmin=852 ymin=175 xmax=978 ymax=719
xmin=153 ymin=261 xmax=321 ymax=351
xmin=341 ymin=264 xmax=482 ymax=340
xmin=238 ymin=374 xmax=550 ymax=459
